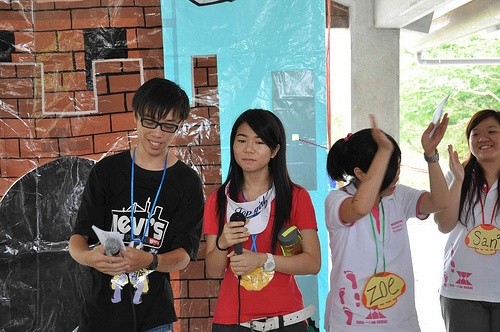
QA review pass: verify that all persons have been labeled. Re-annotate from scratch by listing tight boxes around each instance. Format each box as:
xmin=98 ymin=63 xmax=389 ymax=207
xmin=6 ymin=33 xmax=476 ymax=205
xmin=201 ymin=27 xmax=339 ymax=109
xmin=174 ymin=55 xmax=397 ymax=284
xmin=69 ymin=78 xmax=204 ymax=332
xmin=202 ymin=110 xmax=321 ymax=332
xmin=434 ymin=110 xmax=500 ymax=332
xmin=324 ymin=113 xmax=450 ymax=332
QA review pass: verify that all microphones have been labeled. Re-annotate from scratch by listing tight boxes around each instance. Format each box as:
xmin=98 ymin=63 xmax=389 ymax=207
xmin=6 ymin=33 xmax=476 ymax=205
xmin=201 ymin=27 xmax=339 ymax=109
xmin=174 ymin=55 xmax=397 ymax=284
xmin=230 ymin=212 xmax=246 ymax=256
xmin=103 ymin=238 xmax=123 ymax=259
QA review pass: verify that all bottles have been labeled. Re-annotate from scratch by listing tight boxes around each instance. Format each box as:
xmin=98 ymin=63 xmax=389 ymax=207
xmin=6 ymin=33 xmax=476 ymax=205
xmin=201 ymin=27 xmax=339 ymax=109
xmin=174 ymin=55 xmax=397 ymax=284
xmin=278 ymin=226 xmax=303 ymax=257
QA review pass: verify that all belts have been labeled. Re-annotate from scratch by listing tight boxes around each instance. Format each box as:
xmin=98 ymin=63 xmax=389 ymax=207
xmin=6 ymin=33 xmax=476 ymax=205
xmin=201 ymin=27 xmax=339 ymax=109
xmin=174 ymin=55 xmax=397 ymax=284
xmin=236 ymin=304 xmax=316 ymax=332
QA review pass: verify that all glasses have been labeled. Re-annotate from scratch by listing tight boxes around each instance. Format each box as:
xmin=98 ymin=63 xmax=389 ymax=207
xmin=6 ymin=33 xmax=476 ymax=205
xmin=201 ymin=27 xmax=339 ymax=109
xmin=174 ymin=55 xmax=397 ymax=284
xmin=140 ymin=113 xmax=183 ymax=133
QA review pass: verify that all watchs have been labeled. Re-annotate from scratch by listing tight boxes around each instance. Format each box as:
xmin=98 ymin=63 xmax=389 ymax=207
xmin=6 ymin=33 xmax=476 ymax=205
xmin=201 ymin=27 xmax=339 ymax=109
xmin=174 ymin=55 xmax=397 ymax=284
xmin=264 ymin=253 xmax=275 ymax=271
xmin=146 ymin=253 xmax=158 ymax=270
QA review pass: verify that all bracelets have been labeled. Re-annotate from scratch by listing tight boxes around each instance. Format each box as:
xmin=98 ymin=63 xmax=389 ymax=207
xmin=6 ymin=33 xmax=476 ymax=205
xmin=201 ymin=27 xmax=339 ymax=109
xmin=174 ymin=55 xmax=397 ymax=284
xmin=216 ymin=239 xmax=229 ymax=251
xmin=424 ymin=148 xmax=439 ymax=161
